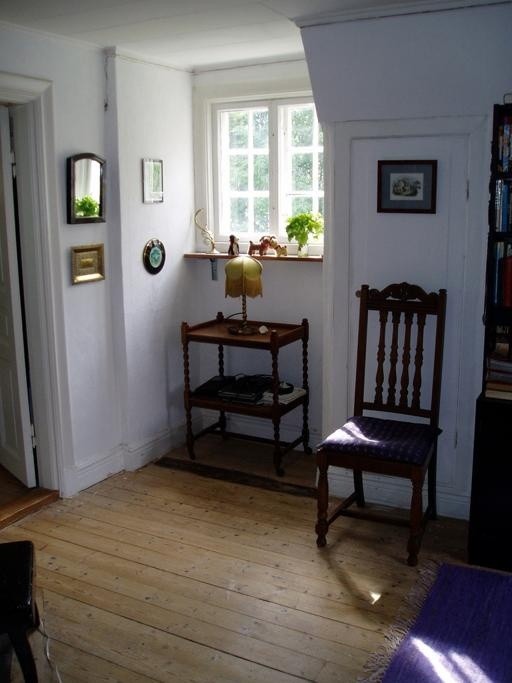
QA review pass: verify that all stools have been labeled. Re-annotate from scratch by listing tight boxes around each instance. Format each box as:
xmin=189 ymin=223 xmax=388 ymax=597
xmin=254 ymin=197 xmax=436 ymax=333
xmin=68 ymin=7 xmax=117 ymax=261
xmin=0 ymin=540 xmax=41 ymax=683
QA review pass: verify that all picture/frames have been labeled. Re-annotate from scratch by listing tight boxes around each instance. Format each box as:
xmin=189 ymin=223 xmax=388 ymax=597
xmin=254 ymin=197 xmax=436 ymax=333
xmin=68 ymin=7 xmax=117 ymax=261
xmin=376 ymin=159 xmax=438 ymax=214
xmin=142 ymin=157 xmax=164 ymax=204
xmin=70 ymin=242 xmax=106 ymax=286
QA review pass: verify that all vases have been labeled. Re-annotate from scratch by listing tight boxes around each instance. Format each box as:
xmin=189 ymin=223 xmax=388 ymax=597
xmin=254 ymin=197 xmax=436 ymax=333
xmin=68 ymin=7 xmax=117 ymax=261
xmin=297 ymin=242 xmax=310 ymax=258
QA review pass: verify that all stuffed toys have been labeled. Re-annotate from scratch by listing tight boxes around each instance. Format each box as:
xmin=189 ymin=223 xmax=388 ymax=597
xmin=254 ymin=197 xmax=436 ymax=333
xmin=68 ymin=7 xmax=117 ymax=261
xmin=228 ymin=234 xmax=288 ymax=256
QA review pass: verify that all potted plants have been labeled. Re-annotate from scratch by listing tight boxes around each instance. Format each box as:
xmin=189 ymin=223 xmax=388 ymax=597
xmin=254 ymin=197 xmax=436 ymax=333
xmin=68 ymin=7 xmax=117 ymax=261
xmin=285 ymin=212 xmax=324 ymax=258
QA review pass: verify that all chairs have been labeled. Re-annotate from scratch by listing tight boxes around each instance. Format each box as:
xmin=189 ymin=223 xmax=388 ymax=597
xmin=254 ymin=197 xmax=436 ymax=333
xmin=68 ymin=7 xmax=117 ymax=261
xmin=315 ymin=282 xmax=447 ymax=566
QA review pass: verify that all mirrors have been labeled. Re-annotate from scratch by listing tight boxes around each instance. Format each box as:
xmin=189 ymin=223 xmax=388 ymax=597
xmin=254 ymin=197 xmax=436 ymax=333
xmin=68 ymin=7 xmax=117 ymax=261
xmin=66 ymin=152 xmax=107 ymax=225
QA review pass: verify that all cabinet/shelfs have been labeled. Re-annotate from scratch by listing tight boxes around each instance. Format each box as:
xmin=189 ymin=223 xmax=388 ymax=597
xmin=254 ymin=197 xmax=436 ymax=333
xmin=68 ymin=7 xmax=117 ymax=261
xmin=180 ymin=311 xmax=313 ymax=478
xmin=466 ymin=103 xmax=512 ymax=573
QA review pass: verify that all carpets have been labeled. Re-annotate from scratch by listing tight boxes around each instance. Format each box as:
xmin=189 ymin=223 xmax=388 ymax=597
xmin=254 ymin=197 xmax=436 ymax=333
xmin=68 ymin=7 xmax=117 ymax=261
xmin=354 ymin=549 xmax=512 ymax=683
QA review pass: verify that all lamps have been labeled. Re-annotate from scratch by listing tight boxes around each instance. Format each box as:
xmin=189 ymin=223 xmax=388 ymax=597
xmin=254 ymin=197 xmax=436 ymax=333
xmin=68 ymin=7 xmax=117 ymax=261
xmin=224 ymin=256 xmax=264 ymax=336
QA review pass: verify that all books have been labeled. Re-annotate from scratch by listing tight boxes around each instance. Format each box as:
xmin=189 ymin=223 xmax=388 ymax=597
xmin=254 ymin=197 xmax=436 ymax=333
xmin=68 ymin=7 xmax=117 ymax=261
xmin=486 ymin=122 xmax=512 ymax=399
xmin=218 ymin=378 xmax=307 ymax=403
